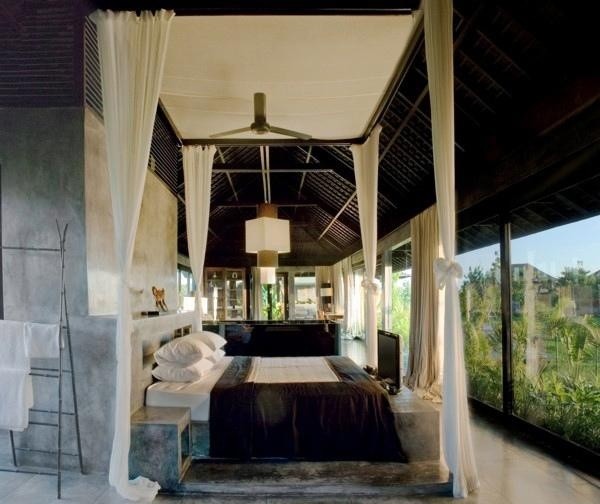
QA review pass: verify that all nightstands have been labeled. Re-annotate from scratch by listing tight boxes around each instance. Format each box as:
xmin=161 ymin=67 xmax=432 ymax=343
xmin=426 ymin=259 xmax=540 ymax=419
xmin=128 ymin=407 xmax=194 ymax=487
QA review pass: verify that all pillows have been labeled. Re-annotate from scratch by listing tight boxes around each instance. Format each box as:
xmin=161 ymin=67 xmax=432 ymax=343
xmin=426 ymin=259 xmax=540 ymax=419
xmin=152 ymin=331 xmax=229 ymax=383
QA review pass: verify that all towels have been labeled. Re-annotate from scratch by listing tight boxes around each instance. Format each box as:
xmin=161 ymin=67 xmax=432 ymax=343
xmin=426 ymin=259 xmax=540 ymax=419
xmin=0 ymin=322 xmax=64 ymax=430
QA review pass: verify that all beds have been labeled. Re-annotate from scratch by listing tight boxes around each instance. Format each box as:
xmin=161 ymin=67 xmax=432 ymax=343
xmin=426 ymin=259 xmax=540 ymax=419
xmin=146 ymin=356 xmax=409 ymax=463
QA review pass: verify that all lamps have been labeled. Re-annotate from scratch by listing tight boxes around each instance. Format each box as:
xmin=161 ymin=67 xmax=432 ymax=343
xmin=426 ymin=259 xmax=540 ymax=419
xmin=245 ymin=202 xmax=291 ymax=289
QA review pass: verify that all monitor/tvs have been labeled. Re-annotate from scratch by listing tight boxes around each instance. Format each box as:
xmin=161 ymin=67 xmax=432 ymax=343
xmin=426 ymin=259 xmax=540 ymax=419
xmin=377 ymin=330 xmax=404 ymax=395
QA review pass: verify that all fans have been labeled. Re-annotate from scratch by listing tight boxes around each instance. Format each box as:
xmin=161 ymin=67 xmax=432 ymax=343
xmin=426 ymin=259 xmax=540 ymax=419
xmin=209 ymin=92 xmax=311 ymax=141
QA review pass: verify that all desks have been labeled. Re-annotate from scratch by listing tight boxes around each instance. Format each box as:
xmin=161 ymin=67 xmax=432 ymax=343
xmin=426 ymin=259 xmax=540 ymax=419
xmin=370 ymin=376 xmax=440 ymax=462
